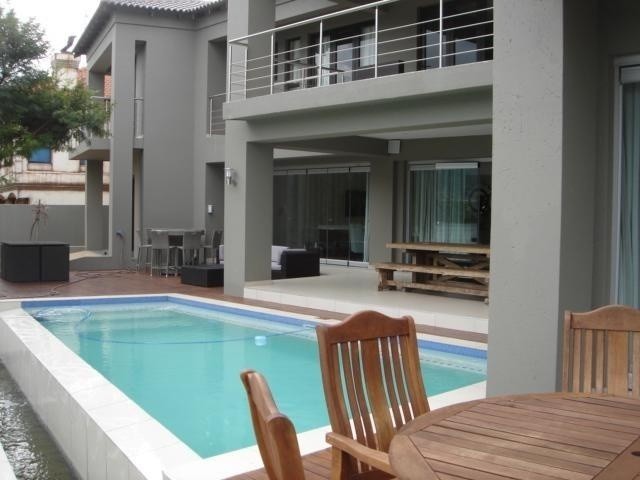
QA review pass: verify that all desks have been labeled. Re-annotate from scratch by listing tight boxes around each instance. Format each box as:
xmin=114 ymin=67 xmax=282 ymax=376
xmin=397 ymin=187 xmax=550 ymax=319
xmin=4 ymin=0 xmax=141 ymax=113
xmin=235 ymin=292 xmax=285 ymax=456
xmin=0 ymin=241 xmax=69 ymax=282
xmin=386 ymin=242 xmax=490 ymax=289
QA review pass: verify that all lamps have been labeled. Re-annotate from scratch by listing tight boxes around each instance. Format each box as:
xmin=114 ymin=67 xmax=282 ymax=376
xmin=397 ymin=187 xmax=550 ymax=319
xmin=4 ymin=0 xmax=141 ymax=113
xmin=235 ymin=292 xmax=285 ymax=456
xmin=225 ymin=168 xmax=234 ymax=184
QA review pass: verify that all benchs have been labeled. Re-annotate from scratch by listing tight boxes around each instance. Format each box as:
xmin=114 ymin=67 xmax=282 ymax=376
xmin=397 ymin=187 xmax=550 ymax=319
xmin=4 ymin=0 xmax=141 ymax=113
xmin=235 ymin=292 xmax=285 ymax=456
xmin=375 ymin=261 xmax=489 ymax=304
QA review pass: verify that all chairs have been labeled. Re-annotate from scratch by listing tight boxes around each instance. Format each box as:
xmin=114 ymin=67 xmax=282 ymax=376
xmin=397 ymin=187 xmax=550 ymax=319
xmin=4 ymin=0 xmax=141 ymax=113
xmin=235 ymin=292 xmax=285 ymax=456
xmin=562 ymin=304 xmax=640 ymax=398
xmin=136 ymin=228 xmax=224 ymax=278
xmin=240 ymin=369 xmax=305 ymax=480
xmin=316 ymin=310 xmax=431 ymax=480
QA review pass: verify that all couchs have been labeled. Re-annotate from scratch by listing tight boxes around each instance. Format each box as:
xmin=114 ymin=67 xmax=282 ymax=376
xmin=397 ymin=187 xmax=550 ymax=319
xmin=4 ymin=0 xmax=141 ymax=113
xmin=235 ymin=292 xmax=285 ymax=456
xmin=219 ymin=244 xmax=320 ymax=279
